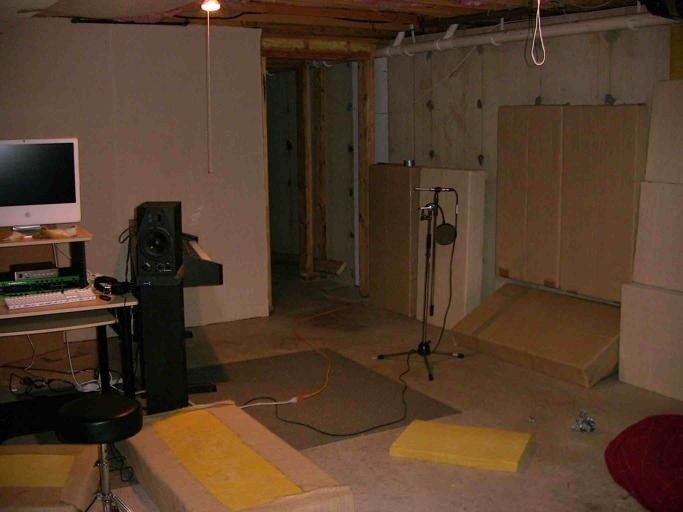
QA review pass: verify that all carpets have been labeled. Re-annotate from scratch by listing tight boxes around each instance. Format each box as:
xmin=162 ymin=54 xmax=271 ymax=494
xmin=262 ymin=271 xmax=395 ymax=336
xmin=187 ymin=345 xmax=462 ymax=452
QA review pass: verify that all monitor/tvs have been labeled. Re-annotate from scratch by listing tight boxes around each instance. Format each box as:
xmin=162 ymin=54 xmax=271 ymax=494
xmin=0 ymin=138 xmax=81 ymax=231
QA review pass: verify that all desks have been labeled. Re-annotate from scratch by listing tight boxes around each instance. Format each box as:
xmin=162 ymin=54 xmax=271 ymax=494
xmin=0 ymin=226 xmax=139 ymax=398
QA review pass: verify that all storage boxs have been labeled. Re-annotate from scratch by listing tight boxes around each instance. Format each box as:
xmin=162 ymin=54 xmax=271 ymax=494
xmin=116 ymin=397 xmax=352 ymax=512
xmin=109 ymin=482 xmax=160 ymax=511
xmin=3 ymin=442 xmax=106 ymax=511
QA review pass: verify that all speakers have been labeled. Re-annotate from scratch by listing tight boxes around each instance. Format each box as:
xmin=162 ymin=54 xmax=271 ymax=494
xmin=133 ymin=286 xmax=188 ymax=415
xmin=136 ymin=201 xmax=182 ymax=288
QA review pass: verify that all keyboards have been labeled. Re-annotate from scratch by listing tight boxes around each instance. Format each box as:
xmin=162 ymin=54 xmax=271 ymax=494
xmin=4 ymin=287 xmax=96 ymax=310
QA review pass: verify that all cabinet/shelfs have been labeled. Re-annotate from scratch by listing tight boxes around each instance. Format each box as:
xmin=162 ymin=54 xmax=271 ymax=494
xmin=366 ymin=164 xmax=488 ymax=332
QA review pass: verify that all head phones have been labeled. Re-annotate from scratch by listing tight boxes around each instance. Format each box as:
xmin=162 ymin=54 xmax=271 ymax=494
xmin=94 ymin=276 xmax=129 ymax=295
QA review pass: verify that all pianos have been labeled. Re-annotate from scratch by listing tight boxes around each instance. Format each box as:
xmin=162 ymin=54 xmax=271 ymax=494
xmin=128 ymin=221 xmax=223 ymax=416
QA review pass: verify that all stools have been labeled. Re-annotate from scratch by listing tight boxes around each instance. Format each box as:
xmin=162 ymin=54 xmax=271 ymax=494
xmin=56 ymin=397 xmax=143 ymax=512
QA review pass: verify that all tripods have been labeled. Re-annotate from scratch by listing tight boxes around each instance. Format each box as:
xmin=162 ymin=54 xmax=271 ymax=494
xmin=378 ymin=204 xmax=464 ymax=381
